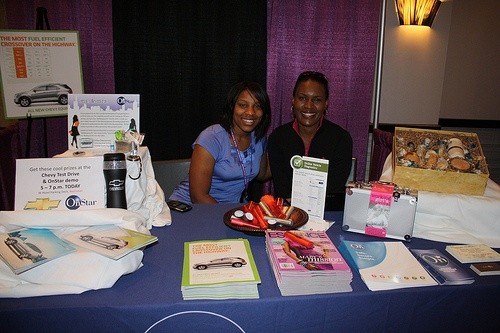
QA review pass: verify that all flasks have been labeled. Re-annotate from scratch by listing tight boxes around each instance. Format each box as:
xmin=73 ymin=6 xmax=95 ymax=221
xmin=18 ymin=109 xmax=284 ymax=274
xmin=103 ymin=152 xmax=128 ymax=209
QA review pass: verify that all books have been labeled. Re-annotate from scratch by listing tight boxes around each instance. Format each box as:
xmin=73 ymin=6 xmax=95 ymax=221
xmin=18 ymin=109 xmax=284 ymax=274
xmin=0 ymin=226 xmax=158 ymax=275
xmin=181 ymin=230 xmax=500 ymax=301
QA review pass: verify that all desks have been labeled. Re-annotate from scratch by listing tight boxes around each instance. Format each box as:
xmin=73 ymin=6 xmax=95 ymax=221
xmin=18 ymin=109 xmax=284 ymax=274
xmin=0 ymin=203 xmax=500 ymax=333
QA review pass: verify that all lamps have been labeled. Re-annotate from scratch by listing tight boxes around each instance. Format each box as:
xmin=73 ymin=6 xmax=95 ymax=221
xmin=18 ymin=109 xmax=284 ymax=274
xmin=394 ymin=0 xmax=443 ymax=28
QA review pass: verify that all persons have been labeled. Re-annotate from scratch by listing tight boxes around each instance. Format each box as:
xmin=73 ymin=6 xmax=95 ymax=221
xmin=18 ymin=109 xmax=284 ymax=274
xmin=169 ymin=82 xmax=272 ymax=205
xmin=268 ymin=71 xmax=353 ymax=210
xmin=282 ymin=230 xmax=335 ymax=271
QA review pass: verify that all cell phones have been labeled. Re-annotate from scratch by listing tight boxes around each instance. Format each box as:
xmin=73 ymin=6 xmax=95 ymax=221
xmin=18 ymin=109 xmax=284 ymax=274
xmin=168 ymin=200 xmax=192 ymax=212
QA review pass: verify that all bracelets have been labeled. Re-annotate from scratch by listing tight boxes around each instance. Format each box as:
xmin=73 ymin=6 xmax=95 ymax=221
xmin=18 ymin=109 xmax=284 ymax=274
xmin=297 ymin=261 xmax=307 ymax=266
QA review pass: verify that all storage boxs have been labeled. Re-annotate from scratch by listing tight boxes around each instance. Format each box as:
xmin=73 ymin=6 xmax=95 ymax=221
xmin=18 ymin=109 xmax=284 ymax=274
xmin=342 ymin=182 xmax=419 ymax=242
xmin=392 ymin=127 xmax=489 ymax=197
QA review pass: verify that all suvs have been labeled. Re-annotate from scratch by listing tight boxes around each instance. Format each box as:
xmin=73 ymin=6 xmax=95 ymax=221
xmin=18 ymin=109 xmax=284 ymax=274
xmin=80 ymin=232 xmax=128 ymax=249
xmin=6 ymin=236 xmax=43 ymax=260
xmin=13 ymin=83 xmax=72 ymax=107
xmin=193 ymin=256 xmax=247 ymax=270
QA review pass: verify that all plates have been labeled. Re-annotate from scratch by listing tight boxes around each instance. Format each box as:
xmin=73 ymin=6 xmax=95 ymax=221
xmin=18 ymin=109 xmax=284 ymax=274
xmin=222 ymin=206 xmax=309 ymax=232
